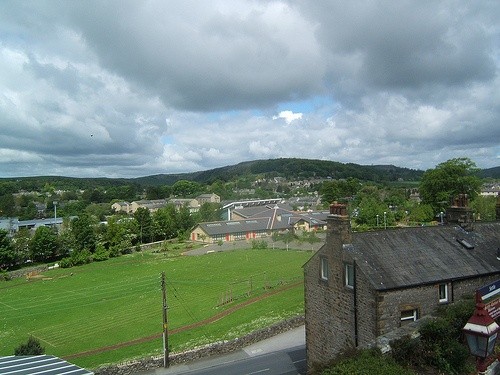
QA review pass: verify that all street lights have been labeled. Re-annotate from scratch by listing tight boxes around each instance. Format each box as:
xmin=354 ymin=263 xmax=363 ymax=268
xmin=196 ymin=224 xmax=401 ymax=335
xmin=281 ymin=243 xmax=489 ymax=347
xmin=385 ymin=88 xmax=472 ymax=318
xmin=463 ymin=286 xmax=500 ymax=375
